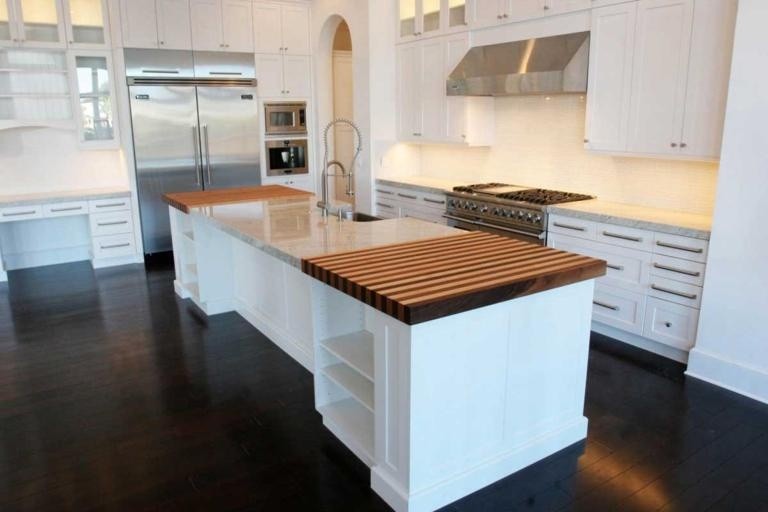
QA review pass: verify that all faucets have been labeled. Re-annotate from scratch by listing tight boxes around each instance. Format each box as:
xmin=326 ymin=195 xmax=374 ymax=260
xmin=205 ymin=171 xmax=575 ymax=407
xmin=315 ymin=157 xmax=349 ymax=217
xmin=322 ymin=114 xmax=363 ymax=217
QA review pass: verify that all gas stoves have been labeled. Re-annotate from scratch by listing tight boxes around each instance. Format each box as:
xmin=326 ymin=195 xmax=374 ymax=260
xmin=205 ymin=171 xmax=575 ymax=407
xmin=452 ymin=181 xmax=597 ymax=212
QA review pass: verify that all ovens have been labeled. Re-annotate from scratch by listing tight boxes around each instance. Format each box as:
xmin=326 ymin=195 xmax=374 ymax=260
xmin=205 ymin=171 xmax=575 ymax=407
xmin=444 ymin=190 xmax=549 ymax=245
xmin=263 ymin=102 xmax=309 ymax=177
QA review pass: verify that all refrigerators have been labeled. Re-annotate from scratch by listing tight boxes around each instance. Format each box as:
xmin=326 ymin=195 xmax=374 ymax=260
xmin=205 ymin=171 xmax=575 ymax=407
xmin=123 ymin=46 xmax=262 ymax=260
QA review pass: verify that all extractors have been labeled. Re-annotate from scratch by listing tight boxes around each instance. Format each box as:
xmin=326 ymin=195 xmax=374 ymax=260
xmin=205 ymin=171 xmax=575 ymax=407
xmin=445 ymin=30 xmax=592 ymax=97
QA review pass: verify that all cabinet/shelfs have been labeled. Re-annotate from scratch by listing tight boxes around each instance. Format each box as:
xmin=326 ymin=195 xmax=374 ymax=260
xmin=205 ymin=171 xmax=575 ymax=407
xmin=120 ymin=0 xmax=310 ymax=54
xmin=376 ymin=184 xmax=447 ymax=225
xmin=313 ymin=277 xmax=389 ymax=469
xmin=547 ymin=215 xmax=709 ymax=356
xmin=1 ymin=1 xmax=122 ymax=151
xmin=255 ymin=54 xmax=317 ymax=194
xmin=1 ymin=198 xmax=138 ymax=282
xmin=169 ymin=205 xmax=234 ymax=315
xmin=583 ymin=1 xmax=738 ymax=163
xmin=392 ymin=0 xmax=627 ymax=45
xmin=393 ymin=32 xmax=496 ymax=147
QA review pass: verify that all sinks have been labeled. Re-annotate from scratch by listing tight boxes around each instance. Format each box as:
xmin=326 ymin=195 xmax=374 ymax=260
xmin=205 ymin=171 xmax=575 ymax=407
xmin=333 ymin=209 xmax=388 ymax=225
